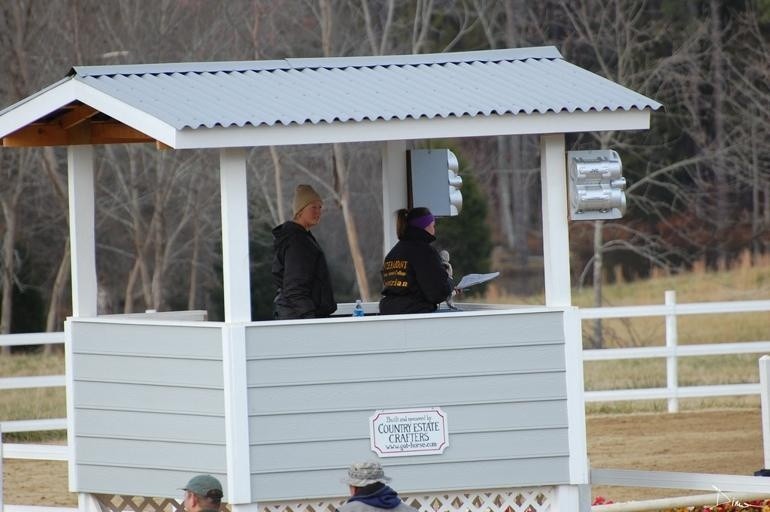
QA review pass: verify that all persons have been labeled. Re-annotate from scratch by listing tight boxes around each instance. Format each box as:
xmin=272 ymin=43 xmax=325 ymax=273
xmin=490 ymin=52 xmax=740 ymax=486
xmin=183 ymin=473 xmax=225 ymax=512
xmin=271 ymin=183 xmax=337 ymax=318
xmin=376 ymin=207 xmax=463 ymax=314
xmin=336 ymin=460 xmax=416 ymax=512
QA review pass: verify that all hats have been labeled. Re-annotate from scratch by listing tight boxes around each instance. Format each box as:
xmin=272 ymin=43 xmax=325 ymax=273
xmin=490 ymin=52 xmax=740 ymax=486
xmin=177 ymin=475 xmax=223 ymax=498
xmin=293 ymin=184 xmax=323 ymax=219
xmin=346 ymin=460 xmax=392 ymax=487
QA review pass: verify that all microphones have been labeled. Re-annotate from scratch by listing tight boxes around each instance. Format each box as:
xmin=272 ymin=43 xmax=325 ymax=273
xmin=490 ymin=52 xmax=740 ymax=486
xmin=439 ymin=249 xmax=449 ymax=264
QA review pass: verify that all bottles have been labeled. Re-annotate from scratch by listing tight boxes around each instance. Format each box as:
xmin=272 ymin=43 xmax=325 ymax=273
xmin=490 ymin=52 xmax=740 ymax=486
xmin=351 ymin=299 xmax=364 ymax=317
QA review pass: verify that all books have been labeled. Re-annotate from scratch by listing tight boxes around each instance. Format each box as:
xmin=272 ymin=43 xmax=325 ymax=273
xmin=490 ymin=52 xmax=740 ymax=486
xmin=455 ymin=270 xmax=500 ymax=290
